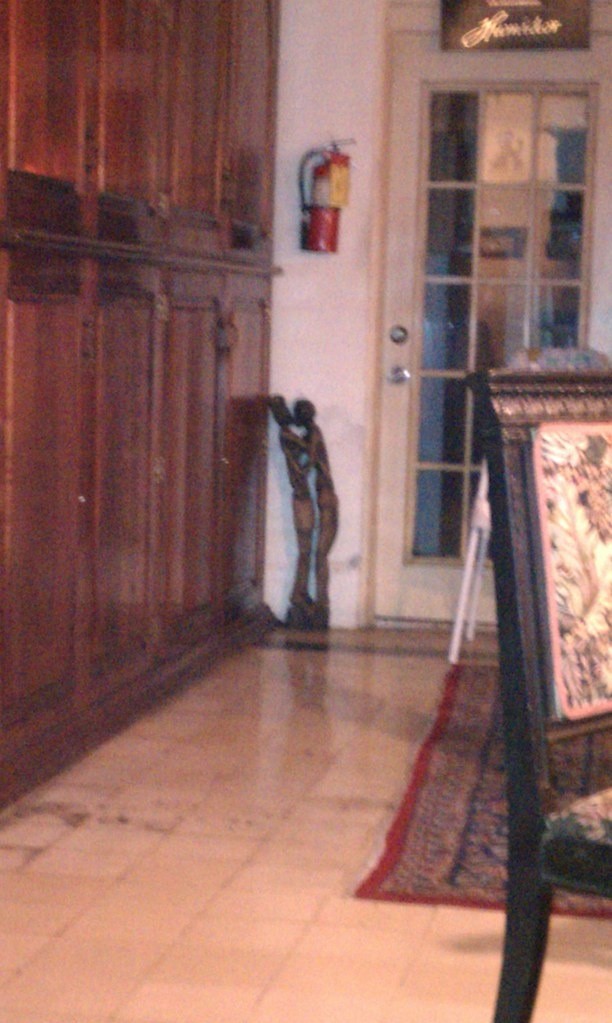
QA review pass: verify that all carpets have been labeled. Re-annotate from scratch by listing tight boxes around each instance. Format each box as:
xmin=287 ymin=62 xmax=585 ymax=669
xmin=357 ymin=662 xmax=611 ymax=921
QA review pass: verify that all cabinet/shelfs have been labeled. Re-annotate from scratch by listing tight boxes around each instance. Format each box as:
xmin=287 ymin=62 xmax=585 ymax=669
xmin=0 ymin=0 xmax=281 ymax=813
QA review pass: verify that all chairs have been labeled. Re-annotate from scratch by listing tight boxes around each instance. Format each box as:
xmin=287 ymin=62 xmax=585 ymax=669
xmin=468 ymin=367 xmax=612 ymax=1023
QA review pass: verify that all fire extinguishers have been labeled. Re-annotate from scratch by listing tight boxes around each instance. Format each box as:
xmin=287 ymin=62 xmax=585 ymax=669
xmin=296 ymin=130 xmax=358 ymax=257
